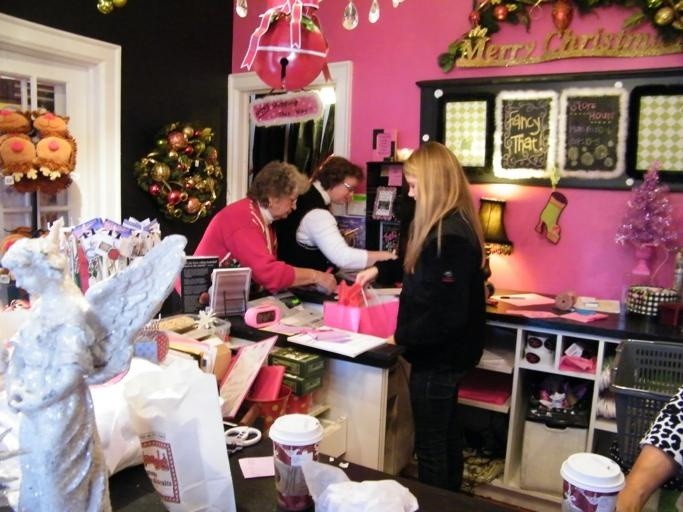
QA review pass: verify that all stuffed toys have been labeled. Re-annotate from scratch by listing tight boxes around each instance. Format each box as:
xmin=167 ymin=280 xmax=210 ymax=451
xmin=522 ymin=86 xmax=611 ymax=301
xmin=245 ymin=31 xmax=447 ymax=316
xmin=0 ymin=105 xmax=79 ymax=198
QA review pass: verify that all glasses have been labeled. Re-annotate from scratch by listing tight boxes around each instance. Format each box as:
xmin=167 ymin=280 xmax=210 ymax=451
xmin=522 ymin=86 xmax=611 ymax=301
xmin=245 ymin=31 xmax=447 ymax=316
xmin=342 ymin=181 xmax=354 ymax=191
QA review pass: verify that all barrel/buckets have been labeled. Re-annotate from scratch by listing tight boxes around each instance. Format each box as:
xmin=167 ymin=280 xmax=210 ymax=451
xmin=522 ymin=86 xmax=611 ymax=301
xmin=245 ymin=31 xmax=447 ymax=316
xmin=243 ymin=384 xmax=292 ymax=431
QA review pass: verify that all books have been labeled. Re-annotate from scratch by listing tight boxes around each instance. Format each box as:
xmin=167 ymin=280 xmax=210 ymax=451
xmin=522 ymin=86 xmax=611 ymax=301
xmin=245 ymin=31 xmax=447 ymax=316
xmin=178 ymin=254 xmax=219 ymax=315
xmin=208 ymin=266 xmax=252 ymax=315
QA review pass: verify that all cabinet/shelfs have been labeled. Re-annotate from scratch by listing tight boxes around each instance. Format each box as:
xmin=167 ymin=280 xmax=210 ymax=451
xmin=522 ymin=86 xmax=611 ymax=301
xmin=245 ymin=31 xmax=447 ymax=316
xmin=458 ymin=320 xmax=621 ymax=510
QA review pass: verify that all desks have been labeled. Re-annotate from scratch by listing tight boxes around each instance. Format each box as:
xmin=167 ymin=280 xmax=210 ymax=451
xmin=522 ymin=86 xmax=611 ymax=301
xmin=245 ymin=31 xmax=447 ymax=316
xmin=0 ymin=419 xmax=538 ymax=512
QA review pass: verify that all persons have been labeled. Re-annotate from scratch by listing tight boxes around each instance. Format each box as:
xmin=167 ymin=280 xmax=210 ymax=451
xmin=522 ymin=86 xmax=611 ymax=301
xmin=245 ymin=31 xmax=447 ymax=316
xmin=353 ymin=141 xmax=488 ymax=491
xmin=0 ymin=226 xmax=114 ymax=511
xmin=171 ymin=161 xmax=340 ymax=314
xmin=612 ymin=384 xmax=683 ymax=512
xmin=274 ymin=155 xmax=400 ymax=275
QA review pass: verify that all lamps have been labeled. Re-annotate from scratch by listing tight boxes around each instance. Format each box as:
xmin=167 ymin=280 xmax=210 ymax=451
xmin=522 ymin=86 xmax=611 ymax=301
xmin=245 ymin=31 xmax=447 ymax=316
xmin=473 ymin=195 xmax=514 ymax=309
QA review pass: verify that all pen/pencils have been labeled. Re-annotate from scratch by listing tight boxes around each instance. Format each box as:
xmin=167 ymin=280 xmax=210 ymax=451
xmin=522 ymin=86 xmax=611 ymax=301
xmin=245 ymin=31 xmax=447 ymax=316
xmin=325 ymin=266 xmax=333 ymax=273
xmin=500 ymin=296 xmax=524 ymax=298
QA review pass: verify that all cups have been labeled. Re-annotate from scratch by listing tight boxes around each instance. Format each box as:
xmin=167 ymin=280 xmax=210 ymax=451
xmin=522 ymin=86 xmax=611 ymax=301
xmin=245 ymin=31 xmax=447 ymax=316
xmin=268 ymin=412 xmax=325 ymax=512
xmin=557 ymin=451 xmax=625 ymax=512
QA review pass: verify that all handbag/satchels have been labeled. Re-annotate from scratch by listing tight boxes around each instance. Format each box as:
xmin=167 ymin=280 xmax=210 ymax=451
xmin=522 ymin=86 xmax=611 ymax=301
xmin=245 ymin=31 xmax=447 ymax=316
xmin=323 ymin=282 xmax=399 ymax=338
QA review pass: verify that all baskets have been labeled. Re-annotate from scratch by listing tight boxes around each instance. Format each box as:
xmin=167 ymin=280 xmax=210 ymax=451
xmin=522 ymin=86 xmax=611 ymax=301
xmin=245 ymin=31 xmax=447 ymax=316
xmin=607 ymin=337 xmax=682 ymax=471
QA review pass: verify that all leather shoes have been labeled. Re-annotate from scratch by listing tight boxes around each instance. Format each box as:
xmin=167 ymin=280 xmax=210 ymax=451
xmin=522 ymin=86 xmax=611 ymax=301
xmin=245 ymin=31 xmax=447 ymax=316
xmin=399 ymin=463 xmax=419 ymax=481
